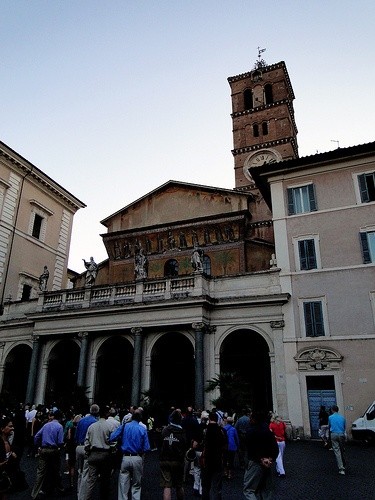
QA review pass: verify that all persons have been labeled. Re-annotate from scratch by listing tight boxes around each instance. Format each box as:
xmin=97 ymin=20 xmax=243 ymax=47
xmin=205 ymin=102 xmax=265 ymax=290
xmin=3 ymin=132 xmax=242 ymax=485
xmin=318 ymin=405 xmax=337 ymax=451
xmin=39 ymin=265 xmax=49 ymax=291
xmin=134 ymin=249 xmax=147 ymax=281
xmin=82 ymin=257 xmax=99 ymax=286
xmin=189 ymin=241 xmax=205 ymax=273
xmin=0 ymin=403 xmax=287 ymax=500
xmin=328 ymin=406 xmax=348 ymax=475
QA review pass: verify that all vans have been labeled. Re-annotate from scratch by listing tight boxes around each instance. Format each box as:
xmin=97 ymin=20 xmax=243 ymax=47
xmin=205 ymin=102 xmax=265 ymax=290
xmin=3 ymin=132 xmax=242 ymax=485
xmin=350 ymin=400 xmax=375 ymax=441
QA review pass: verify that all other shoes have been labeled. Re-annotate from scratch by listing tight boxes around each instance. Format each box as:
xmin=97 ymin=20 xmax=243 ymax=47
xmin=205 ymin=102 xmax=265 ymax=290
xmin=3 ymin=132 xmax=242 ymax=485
xmin=57 ymin=484 xmax=64 ymax=491
xmin=280 ymin=474 xmax=285 ymax=480
xmin=339 ymin=471 xmax=345 ymax=474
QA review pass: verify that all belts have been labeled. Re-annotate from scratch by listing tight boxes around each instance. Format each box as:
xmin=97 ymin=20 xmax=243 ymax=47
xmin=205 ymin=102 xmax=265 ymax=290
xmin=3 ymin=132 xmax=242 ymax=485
xmin=124 ymin=453 xmax=142 ymax=456
xmin=41 ymin=446 xmax=56 ymax=449
xmin=91 ymin=448 xmax=114 ymax=452
